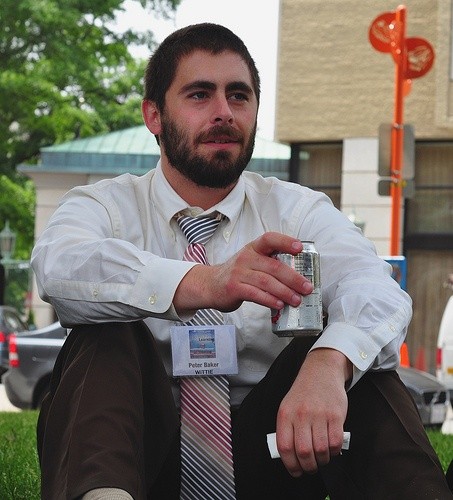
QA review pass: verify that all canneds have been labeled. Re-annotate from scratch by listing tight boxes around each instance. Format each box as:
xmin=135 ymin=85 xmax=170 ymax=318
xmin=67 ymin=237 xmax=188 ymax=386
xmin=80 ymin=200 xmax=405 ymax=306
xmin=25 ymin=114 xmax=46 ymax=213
xmin=271 ymin=239 xmax=325 ymax=335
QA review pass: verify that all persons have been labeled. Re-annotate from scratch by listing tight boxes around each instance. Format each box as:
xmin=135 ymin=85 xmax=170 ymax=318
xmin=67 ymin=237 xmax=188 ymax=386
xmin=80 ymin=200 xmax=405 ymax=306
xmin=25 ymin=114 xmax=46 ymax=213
xmin=27 ymin=24 xmax=453 ymax=500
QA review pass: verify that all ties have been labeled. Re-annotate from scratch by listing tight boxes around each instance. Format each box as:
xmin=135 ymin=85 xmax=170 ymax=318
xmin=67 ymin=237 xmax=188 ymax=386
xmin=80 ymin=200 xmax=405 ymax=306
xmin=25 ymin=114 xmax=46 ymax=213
xmin=174 ymin=212 xmax=238 ymax=500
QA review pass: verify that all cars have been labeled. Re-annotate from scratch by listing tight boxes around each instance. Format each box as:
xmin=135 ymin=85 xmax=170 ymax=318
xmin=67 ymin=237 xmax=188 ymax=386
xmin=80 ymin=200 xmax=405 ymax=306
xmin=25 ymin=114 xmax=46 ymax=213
xmin=3 ymin=321 xmax=446 ymax=431
